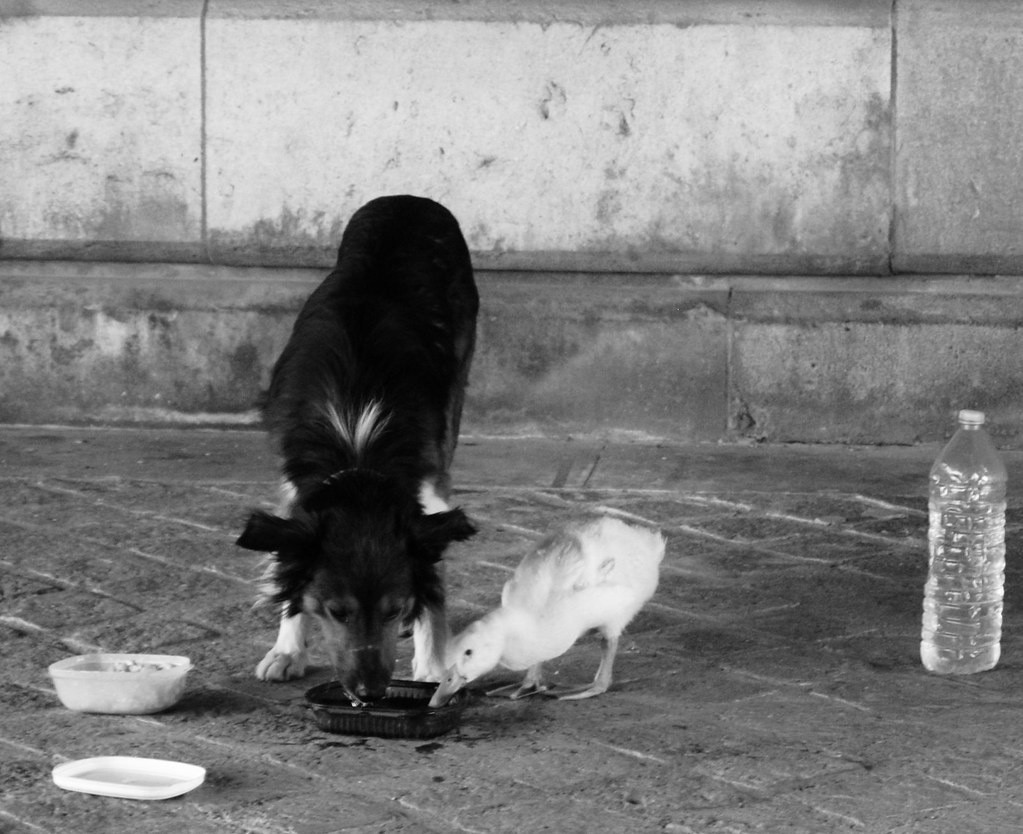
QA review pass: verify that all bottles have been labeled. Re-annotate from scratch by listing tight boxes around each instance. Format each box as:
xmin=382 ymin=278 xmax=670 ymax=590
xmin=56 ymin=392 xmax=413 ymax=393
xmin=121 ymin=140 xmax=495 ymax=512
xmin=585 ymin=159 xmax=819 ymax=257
xmin=921 ymin=411 xmax=1007 ymax=675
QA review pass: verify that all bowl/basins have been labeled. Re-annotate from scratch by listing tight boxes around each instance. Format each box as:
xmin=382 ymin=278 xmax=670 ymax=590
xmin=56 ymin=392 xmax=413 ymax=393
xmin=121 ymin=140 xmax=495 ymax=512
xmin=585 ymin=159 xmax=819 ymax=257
xmin=306 ymin=680 xmax=472 ymax=738
xmin=48 ymin=653 xmax=194 ymax=713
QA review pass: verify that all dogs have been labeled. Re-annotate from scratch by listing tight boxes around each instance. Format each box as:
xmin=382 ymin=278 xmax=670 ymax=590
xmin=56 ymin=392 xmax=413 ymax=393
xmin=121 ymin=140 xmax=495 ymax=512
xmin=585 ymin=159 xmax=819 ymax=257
xmin=233 ymin=194 xmax=480 ymax=707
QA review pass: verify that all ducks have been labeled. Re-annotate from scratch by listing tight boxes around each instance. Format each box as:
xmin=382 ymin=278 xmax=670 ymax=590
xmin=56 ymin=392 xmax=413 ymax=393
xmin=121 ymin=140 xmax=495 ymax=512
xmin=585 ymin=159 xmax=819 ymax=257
xmin=429 ymin=515 xmax=666 ymax=709
